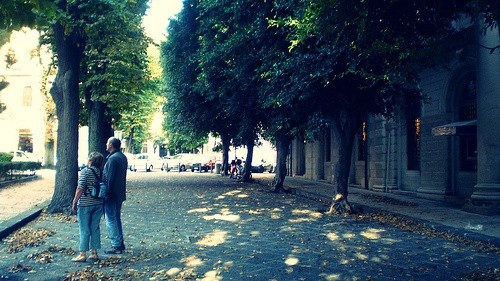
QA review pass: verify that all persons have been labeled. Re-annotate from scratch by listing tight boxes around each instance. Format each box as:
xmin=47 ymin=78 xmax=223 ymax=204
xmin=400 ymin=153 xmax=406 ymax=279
xmin=72 ymin=152 xmax=107 ymax=262
xmin=230 ymin=157 xmax=239 ymax=179
xmin=101 ymin=135 xmax=128 ymax=254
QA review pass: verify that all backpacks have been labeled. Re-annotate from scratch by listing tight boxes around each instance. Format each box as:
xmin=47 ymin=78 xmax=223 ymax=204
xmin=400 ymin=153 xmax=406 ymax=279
xmin=84 ymin=168 xmax=106 ymax=199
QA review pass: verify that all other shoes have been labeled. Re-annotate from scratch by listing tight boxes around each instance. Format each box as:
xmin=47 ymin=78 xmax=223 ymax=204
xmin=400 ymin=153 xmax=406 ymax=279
xmin=71 ymin=255 xmax=86 ymax=262
xmin=104 ymin=247 xmax=122 ymax=254
xmin=111 ymin=244 xmax=125 ymax=250
xmin=86 ymin=256 xmax=98 ymax=262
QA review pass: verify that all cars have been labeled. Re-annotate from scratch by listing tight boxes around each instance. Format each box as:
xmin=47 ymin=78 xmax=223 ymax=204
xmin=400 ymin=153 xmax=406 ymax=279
xmin=6 ymin=150 xmax=36 ymax=162
xmin=191 ymin=156 xmax=218 ymax=172
xmin=165 ymin=153 xmax=205 ymax=172
xmin=127 ymin=153 xmax=165 ymax=172
xmin=251 ymin=159 xmax=273 ymax=173
